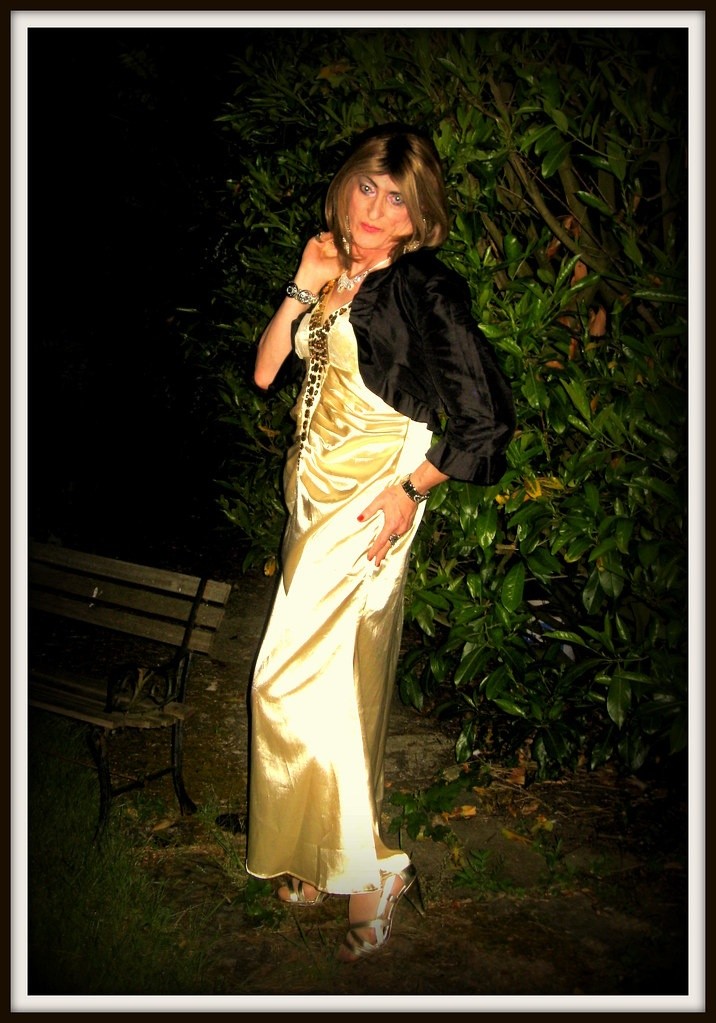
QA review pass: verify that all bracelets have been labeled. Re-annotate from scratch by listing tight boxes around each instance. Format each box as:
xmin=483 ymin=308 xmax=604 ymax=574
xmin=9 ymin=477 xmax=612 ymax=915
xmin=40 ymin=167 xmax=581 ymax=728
xmin=400 ymin=473 xmax=431 ymax=503
xmin=284 ymin=281 xmax=319 ymax=305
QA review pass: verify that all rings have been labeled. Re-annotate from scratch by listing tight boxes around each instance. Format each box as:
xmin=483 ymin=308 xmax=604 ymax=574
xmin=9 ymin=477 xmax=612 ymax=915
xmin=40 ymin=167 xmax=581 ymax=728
xmin=389 ymin=533 xmax=400 ymax=545
xmin=316 ymin=232 xmax=324 ymax=238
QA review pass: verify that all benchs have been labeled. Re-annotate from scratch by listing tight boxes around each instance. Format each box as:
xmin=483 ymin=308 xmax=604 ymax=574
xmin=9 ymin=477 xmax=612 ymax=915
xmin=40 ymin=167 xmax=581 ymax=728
xmin=28 ymin=544 xmax=232 ymax=848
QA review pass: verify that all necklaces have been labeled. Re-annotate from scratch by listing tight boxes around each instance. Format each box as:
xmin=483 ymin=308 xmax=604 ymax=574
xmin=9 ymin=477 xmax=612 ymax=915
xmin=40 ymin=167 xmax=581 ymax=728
xmin=337 ymin=237 xmax=420 ymax=292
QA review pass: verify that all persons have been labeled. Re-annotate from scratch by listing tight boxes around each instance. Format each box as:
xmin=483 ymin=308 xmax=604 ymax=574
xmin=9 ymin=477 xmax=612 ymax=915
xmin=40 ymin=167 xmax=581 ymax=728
xmin=244 ymin=132 xmax=518 ymax=967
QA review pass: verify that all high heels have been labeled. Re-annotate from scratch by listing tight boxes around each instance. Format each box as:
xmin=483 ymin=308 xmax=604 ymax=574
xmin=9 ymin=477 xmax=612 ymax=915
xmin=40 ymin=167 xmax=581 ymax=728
xmin=331 ymin=862 xmax=427 ymax=966
xmin=279 ymin=875 xmax=334 ymax=907
